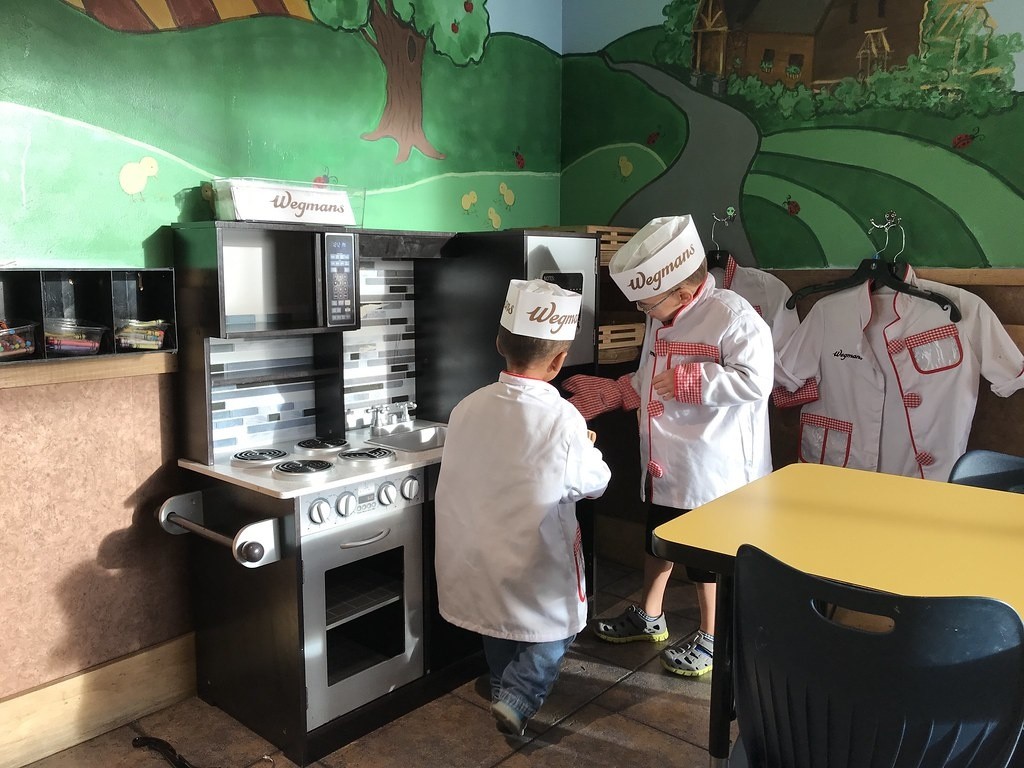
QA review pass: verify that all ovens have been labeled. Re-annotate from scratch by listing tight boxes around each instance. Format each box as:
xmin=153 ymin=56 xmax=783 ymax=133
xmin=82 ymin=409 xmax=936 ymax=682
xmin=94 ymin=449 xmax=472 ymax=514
xmin=287 ymin=463 xmax=428 ymax=733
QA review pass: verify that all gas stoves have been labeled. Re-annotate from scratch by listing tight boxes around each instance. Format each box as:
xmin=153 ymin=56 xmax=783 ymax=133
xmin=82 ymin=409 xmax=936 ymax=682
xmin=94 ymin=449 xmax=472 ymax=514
xmin=227 ymin=434 xmax=399 ymax=479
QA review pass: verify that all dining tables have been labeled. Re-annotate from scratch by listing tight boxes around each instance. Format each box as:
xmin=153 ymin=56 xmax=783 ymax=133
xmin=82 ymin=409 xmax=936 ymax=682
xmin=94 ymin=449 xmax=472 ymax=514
xmin=651 ymin=462 xmax=1024 ymax=768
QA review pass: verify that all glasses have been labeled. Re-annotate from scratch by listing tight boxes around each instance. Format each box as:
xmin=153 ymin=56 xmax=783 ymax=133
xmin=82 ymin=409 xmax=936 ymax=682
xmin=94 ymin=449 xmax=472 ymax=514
xmin=636 ymin=293 xmax=672 ymax=314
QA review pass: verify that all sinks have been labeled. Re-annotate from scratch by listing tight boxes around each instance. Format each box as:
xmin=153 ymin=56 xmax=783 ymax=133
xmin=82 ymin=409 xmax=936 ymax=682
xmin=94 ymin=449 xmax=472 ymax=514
xmin=364 ymin=423 xmax=450 ymax=454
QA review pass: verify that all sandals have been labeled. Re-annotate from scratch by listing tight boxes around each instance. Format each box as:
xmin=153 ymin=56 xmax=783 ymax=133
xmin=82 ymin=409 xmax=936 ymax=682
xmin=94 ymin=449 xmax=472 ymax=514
xmin=660 ymin=629 xmax=725 ymax=677
xmin=592 ymin=603 xmax=670 ymax=643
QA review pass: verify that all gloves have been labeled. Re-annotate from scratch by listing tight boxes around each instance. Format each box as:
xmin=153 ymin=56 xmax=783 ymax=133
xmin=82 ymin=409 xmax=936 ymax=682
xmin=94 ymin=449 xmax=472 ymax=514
xmin=561 ymin=373 xmax=641 ymax=421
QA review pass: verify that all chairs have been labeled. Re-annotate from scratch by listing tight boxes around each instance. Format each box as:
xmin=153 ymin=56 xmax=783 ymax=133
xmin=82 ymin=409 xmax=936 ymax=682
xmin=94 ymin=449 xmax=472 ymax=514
xmin=728 ymin=543 xmax=1024 ymax=768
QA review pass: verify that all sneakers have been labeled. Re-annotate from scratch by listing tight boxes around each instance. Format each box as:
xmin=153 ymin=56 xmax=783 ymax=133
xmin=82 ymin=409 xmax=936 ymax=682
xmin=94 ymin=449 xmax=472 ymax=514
xmin=489 ymin=696 xmax=530 ymax=738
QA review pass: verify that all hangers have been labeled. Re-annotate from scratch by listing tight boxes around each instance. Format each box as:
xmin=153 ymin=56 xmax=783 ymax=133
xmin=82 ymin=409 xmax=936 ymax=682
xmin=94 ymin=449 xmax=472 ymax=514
xmin=705 ymin=217 xmax=729 ymax=272
xmin=786 ymin=224 xmax=961 ymax=322
xmin=870 ymin=224 xmax=906 ymax=293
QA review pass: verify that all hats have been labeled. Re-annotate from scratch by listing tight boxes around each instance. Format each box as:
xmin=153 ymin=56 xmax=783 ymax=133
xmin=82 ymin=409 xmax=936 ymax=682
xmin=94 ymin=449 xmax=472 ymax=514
xmin=499 ymin=279 xmax=582 ymax=341
xmin=609 ymin=214 xmax=706 ymax=302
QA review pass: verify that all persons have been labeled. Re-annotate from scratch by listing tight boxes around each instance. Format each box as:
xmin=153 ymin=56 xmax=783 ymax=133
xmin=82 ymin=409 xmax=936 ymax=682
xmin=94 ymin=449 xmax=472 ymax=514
xmin=433 ymin=278 xmax=611 ymax=737
xmin=561 ymin=213 xmax=774 ymax=676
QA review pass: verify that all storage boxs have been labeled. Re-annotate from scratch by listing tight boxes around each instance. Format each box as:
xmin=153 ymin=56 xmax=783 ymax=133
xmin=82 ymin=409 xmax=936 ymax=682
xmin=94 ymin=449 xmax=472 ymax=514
xmin=521 ymin=224 xmax=645 ymax=350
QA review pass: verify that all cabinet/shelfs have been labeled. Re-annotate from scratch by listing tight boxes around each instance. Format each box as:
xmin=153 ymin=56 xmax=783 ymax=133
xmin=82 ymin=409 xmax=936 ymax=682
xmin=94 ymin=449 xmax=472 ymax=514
xmin=0 ymin=267 xmax=178 ymax=366
